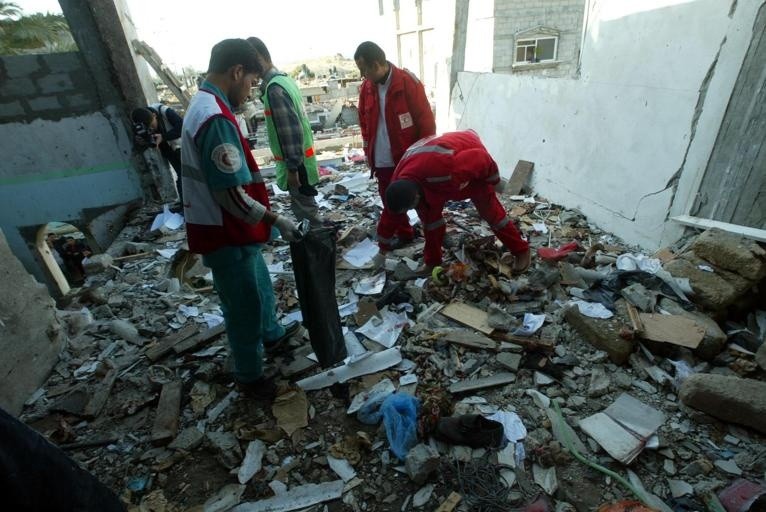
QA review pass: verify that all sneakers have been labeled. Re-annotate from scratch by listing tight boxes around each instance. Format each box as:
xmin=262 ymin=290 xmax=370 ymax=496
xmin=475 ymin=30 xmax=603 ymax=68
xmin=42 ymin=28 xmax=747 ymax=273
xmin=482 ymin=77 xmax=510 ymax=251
xmin=262 ymin=321 xmax=301 ymax=352
xmin=234 ymin=378 xmax=275 ymax=398
xmin=512 ymin=247 xmax=531 ymax=275
xmin=415 ymin=263 xmax=432 ymax=276
xmin=434 ymin=415 xmax=504 ymax=447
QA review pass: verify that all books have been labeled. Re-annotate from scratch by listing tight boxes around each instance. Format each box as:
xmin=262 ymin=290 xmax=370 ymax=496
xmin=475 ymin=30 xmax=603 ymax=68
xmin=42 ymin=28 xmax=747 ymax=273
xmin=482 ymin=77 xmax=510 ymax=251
xmin=581 ymin=394 xmax=670 ymax=465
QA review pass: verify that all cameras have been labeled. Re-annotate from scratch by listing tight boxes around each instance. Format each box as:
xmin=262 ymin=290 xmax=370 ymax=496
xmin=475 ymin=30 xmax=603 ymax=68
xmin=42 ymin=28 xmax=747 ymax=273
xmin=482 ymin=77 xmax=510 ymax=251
xmin=132 ymin=120 xmax=157 ymax=148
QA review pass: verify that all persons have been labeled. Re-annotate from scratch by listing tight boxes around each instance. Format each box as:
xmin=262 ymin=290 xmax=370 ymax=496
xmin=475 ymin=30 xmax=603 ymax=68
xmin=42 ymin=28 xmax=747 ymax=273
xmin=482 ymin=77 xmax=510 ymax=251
xmin=128 ymin=102 xmax=184 ymax=201
xmin=182 ymin=41 xmax=310 ymax=387
xmin=247 ymin=34 xmax=325 ymax=225
xmin=380 ymin=127 xmax=532 ymax=277
xmin=353 ymin=42 xmax=439 ymax=241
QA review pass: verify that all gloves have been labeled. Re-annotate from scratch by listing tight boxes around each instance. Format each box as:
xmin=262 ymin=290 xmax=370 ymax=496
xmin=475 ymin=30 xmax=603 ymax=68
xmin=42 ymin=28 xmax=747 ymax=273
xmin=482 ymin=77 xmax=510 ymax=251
xmin=495 ymin=180 xmax=505 ymax=193
xmin=371 ymin=254 xmax=385 ymax=276
xmin=273 ymin=215 xmax=306 ymax=243
